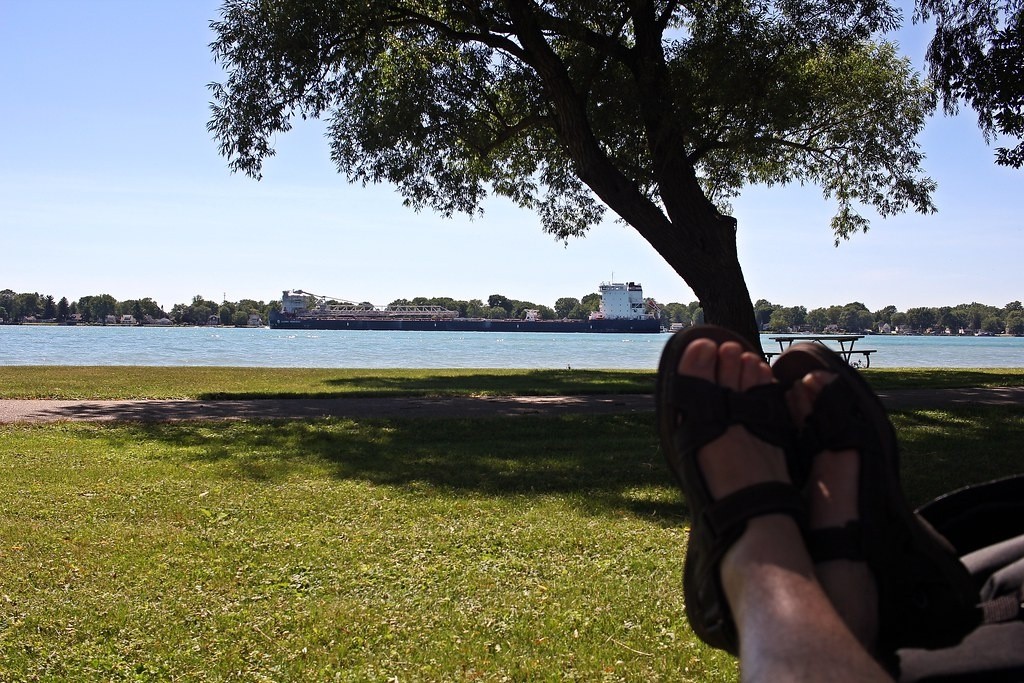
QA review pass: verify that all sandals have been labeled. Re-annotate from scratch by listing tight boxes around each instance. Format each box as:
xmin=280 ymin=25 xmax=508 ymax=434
xmin=654 ymin=321 xmax=983 ymax=659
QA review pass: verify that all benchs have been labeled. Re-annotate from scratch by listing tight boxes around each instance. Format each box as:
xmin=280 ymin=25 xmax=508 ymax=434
xmin=766 ymin=350 xmax=876 ymax=368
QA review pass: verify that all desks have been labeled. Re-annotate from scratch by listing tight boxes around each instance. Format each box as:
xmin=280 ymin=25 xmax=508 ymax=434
xmin=768 ymin=335 xmax=864 ymax=363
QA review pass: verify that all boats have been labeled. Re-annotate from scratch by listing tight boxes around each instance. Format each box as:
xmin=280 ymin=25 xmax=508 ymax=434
xmin=268 ymin=282 xmax=673 ymax=333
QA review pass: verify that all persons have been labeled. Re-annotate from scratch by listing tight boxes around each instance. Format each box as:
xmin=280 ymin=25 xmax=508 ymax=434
xmin=655 ymin=325 xmax=984 ymax=681
